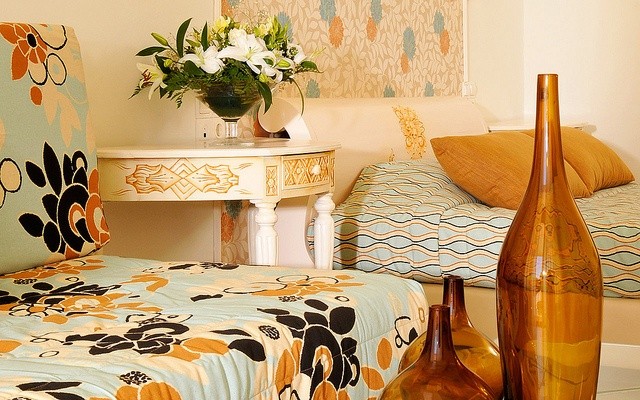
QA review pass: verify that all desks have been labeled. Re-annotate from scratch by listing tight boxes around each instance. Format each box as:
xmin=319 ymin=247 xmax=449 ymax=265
xmin=96 ymin=136 xmax=339 ymax=269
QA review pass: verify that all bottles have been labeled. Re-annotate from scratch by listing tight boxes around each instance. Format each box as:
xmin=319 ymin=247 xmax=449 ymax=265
xmin=496 ymin=73 xmax=603 ymax=400
xmin=377 ymin=305 xmax=495 ymax=399
xmin=398 ymin=275 xmax=504 ymax=400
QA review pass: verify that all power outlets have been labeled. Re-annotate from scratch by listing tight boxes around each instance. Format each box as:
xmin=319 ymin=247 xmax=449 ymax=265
xmin=462 ymin=81 xmax=478 ymax=100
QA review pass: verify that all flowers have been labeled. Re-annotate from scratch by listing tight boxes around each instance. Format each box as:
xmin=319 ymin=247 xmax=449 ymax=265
xmin=128 ymin=17 xmax=329 ymax=119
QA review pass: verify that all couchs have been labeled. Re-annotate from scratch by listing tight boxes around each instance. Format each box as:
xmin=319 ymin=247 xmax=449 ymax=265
xmin=0 ymin=23 xmax=435 ymax=399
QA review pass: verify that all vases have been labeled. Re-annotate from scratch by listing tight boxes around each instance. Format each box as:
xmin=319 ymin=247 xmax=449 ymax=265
xmin=182 ymin=80 xmax=282 ymax=147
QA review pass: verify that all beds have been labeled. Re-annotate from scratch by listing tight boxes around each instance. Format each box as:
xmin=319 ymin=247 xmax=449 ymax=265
xmin=247 ymin=93 xmax=639 ymax=372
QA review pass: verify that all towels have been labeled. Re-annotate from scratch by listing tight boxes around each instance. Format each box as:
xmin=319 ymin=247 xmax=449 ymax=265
xmin=428 ymin=131 xmax=592 ymax=214
xmin=520 ymin=124 xmax=635 ymax=194
